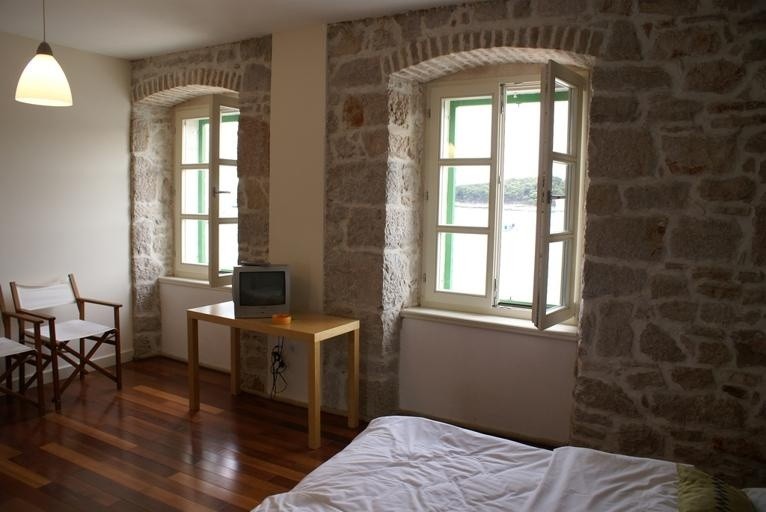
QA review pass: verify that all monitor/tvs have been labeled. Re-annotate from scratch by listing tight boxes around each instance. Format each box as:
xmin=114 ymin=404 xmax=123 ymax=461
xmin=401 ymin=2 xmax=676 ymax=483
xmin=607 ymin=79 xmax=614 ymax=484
xmin=232 ymin=264 xmax=290 ymax=318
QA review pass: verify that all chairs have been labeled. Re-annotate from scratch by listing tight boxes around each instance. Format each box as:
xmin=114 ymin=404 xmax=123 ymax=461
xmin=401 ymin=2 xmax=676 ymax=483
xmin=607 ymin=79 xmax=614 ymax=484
xmin=0 ymin=273 xmax=123 ymax=417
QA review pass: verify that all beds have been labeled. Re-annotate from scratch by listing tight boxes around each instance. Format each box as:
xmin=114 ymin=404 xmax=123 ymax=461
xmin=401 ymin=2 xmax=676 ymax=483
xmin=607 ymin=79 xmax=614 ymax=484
xmin=248 ymin=415 xmax=766 ymax=512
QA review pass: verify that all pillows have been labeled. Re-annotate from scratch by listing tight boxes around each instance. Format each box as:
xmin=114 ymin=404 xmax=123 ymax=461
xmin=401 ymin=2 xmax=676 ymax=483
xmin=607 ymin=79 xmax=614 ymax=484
xmin=677 ymin=461 xmax=758 ymax=512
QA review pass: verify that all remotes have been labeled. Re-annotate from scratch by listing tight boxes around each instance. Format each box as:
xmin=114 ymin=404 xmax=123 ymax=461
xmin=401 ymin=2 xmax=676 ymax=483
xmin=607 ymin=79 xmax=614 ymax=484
xmin=241 ymin=261 xmax=265 ymax=266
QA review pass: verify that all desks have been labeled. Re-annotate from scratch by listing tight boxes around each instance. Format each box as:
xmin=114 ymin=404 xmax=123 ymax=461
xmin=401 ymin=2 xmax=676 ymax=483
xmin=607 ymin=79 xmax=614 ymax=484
xmin=184 ymin=300 xmax=360 ymax=450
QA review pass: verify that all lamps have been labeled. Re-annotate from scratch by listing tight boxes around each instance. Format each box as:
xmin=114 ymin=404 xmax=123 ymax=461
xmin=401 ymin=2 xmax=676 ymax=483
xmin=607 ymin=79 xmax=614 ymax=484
xmin=15 ymin=1 xmax=73 ymax=107
